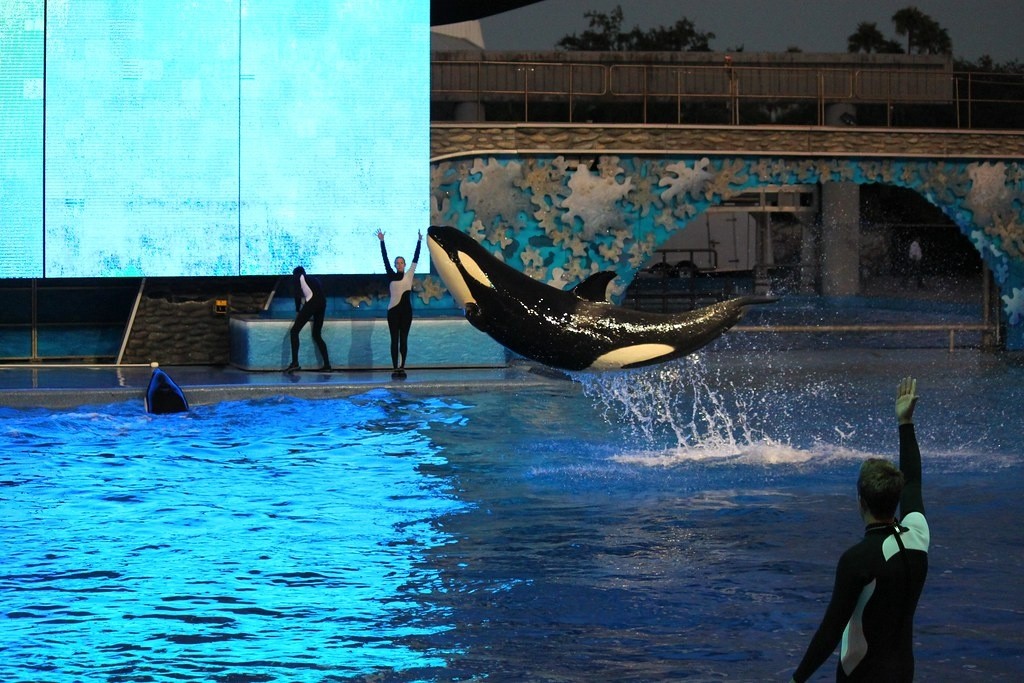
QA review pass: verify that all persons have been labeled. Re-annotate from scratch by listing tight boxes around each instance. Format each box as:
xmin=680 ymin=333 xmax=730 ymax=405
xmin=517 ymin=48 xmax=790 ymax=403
xmin=902 ymin=235 xmax=927 ymax=290
xmin=373 ymin=228 xmax=423 ymax=379
xmin=285 ymin=266 xmax=331 ymax=373
xmin=789 ymin=376 xmax=930 ymax=683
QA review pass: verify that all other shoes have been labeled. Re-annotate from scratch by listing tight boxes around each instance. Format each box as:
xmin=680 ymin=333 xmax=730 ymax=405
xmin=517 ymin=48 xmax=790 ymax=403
xmin=319 ymin=365 xmax=331 ymax=372
xmin=284 ymin=363 xmax=300 ymax=375
xmin=392 ymin=368 xmax=399 ymax=377
xmin=399 ymin=367 xmax=407 ymax=377
